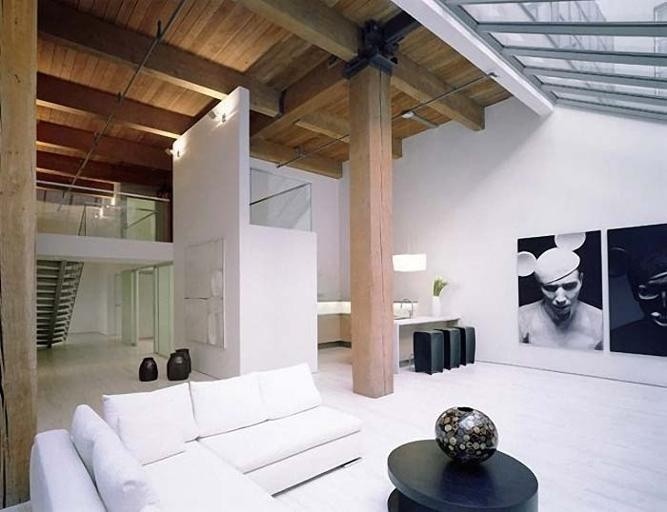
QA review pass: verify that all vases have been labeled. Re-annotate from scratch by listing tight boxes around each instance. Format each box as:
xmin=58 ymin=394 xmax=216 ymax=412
xmin=175 ymin=348 xmax=192 ymax=374
xmin=138 ymin=357 xmax=159 ymax=383
xmin=434 ymin=405 xmax=498 ymax=465
xmin=166 ymin=353 xmax=189 ymax=381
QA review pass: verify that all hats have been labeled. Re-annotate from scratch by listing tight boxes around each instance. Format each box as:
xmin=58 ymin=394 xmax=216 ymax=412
xmin=609 ymin=246 xmax=667 ymax=286
xmin=517 ymin=233 xmax=586 ymax=284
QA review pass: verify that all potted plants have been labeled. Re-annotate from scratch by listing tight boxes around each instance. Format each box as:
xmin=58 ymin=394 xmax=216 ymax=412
xmin=431 ymin=278 xmax=450 ymax=316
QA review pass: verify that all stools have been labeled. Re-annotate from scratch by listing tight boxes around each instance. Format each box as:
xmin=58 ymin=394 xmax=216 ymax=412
xmin=434 ymin=327 xmax=462 ymax=371
xmin=413 ymin=330 xmax=445 ymax=375
xmin=451 ymin=326 xmax=476 ymax=366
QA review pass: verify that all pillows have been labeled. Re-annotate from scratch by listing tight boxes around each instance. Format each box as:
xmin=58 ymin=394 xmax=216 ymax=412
xmin=100 ymin=382 xmax=186 ymax=464
xmin=68 ymin=403 xmax=114 ymax=476
xmin=258 ymin=362 xmax=323 ymax=421
xmin=91 ymin=425 xmax=163 ymax=512
xmin=189 ymin=370 xmax=268 ymax=438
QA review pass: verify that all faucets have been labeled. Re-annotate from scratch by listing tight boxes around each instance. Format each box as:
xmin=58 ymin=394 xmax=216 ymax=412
xmin=400 ymin=299 xmax=414 ymax=319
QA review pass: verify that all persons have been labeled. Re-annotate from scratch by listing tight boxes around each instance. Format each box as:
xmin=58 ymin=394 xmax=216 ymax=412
xmin=517 ymin=232 xmax=604 ymax=351
xmin=607 ymin=238 xmax=667 ymax=357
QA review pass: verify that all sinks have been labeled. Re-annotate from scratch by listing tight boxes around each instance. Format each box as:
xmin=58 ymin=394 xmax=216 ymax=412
xmin=394 ymin=317 xmax=409 ymax=320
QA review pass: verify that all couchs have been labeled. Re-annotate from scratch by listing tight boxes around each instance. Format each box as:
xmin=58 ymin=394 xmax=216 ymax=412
xmin=189 ymin=362 xmax=363 ymax=498
xmin=28 ymin=427 xmax=283 ymax=512
